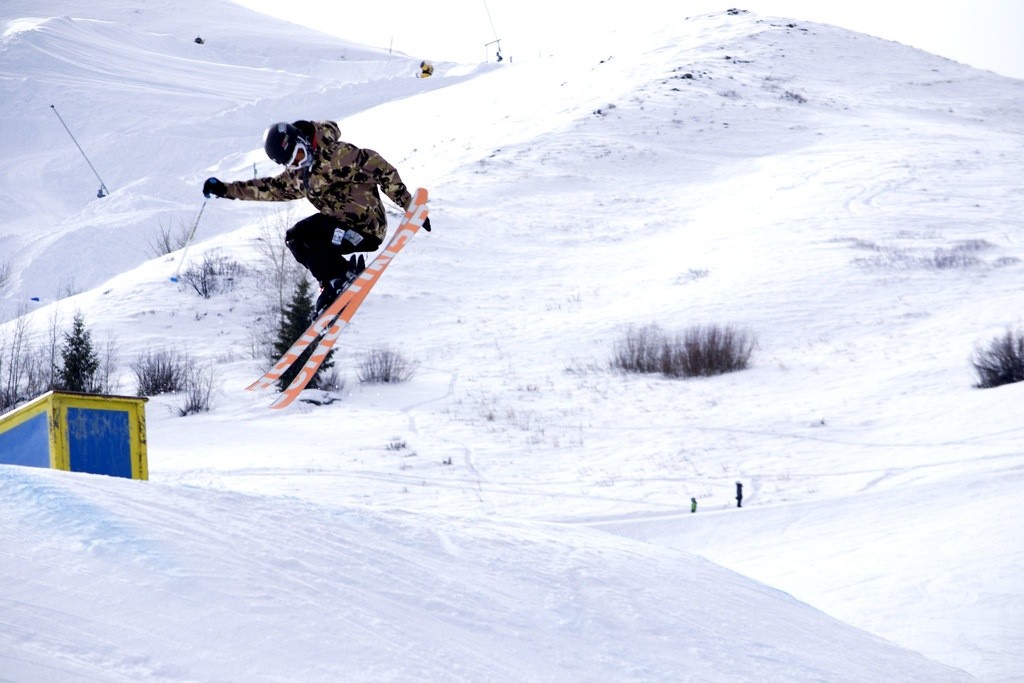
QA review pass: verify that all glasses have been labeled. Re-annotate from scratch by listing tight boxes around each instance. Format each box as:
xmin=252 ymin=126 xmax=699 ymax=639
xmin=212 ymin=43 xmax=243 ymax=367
xmin=287 ymin=130 xmax=308 ymax=170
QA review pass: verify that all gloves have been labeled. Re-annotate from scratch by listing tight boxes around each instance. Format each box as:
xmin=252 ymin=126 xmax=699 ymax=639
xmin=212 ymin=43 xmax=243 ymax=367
xmin=203 ymin=177 xmax=226 ymax=198
xmin=422 ymin=217 xmax=432 ymax=232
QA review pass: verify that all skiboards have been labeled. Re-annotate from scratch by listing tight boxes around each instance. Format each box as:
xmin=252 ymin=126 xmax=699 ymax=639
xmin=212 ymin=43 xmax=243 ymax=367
xmin=244 ymin=188 xmax=428 ymax=410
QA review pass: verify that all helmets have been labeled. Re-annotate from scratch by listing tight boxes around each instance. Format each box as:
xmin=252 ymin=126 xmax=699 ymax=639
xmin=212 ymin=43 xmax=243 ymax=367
xmin=262 ymin=121 xmax=312 ymax=171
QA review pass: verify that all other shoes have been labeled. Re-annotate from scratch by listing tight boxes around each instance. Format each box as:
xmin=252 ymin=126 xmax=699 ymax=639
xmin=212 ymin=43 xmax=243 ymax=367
xmin=316 ymin=264 xmax=358 ymax=310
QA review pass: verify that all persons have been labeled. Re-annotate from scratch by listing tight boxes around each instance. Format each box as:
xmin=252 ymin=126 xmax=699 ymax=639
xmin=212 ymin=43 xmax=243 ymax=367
xmin=691 ymin=498 xmax=697 ymax=512
xmin=203 ymin=121 xmax=430 ymax=329
xmin=734 ymin=480 xmax=743 ymax=507
